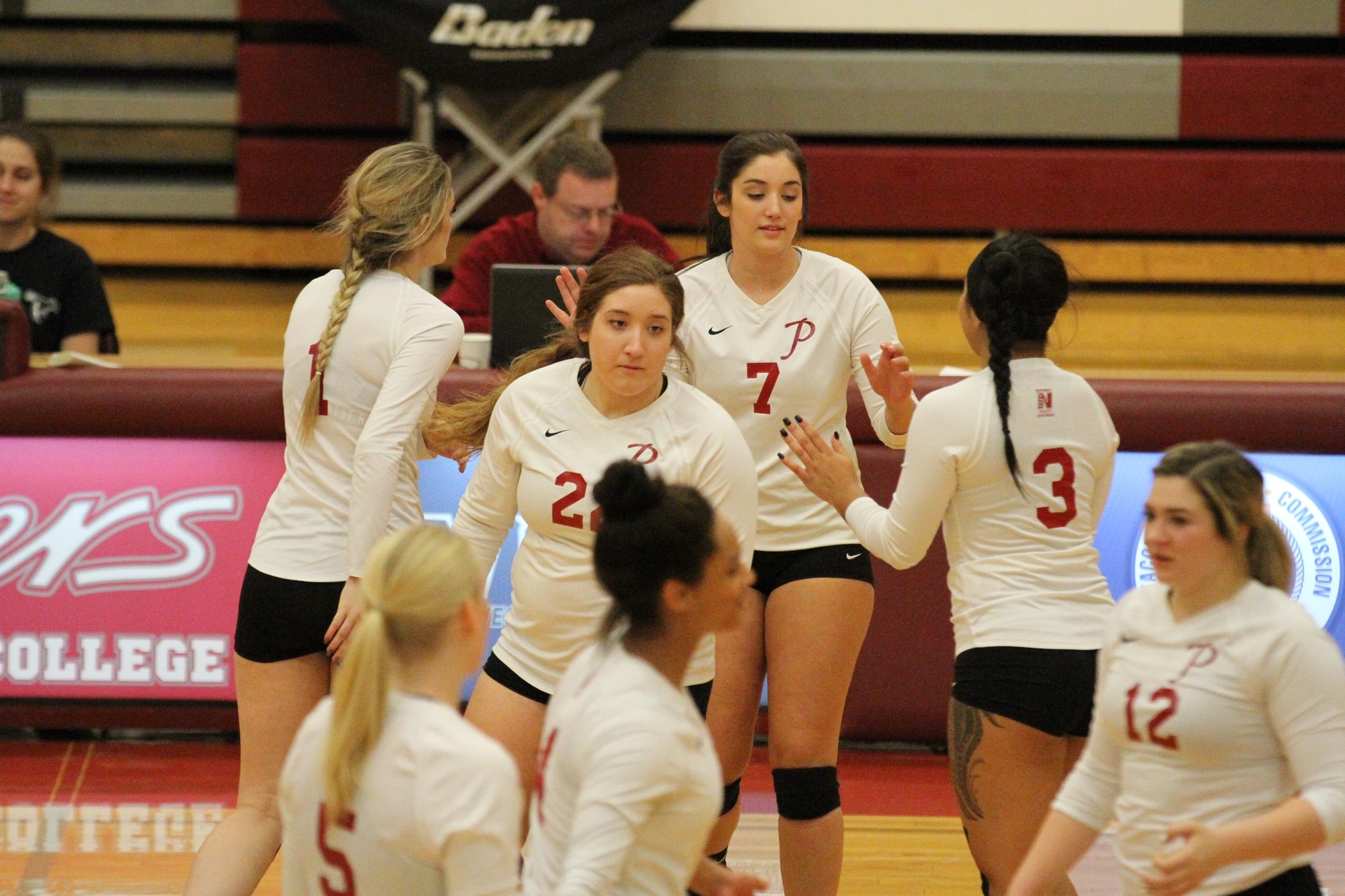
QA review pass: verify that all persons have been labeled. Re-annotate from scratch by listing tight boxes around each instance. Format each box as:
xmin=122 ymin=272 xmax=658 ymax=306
xmin=0 ymin=120 xmax=122 ymax=358
xmin=777 ymin=232 xmax=1120 ymax=895
xmin=436 ymin=133 xmax=685 ymax=333
xmin=278 ymin=520 xmax=524 ymax=896
xmin=1007 ymin=442 xmax=1345 ymax=895
xmin=182 ymin=142 xmax=473 ymax=896
xmin=517 ymin=459 xmax=768 ymax=896
xmin=424 ymin=247 xmax=758 ymax=831
xmin=543 ymin=131 xmax=919 ymax=896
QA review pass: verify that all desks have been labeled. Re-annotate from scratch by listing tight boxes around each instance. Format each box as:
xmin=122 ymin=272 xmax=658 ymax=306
xmin=0 ymin=366 xmax=1345 ymax=741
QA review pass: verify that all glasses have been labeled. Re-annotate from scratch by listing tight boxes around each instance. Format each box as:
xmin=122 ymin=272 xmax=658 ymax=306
xmin=551 ymin=197 xmax=624 ymax=227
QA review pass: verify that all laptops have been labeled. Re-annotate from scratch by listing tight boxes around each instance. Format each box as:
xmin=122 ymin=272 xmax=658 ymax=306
xmin=492 ymin=263 xmax=593 ymax=369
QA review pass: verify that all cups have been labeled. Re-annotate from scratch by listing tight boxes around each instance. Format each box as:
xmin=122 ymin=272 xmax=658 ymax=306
xmin=458 ymin=332 xmax=493 ymax=371
